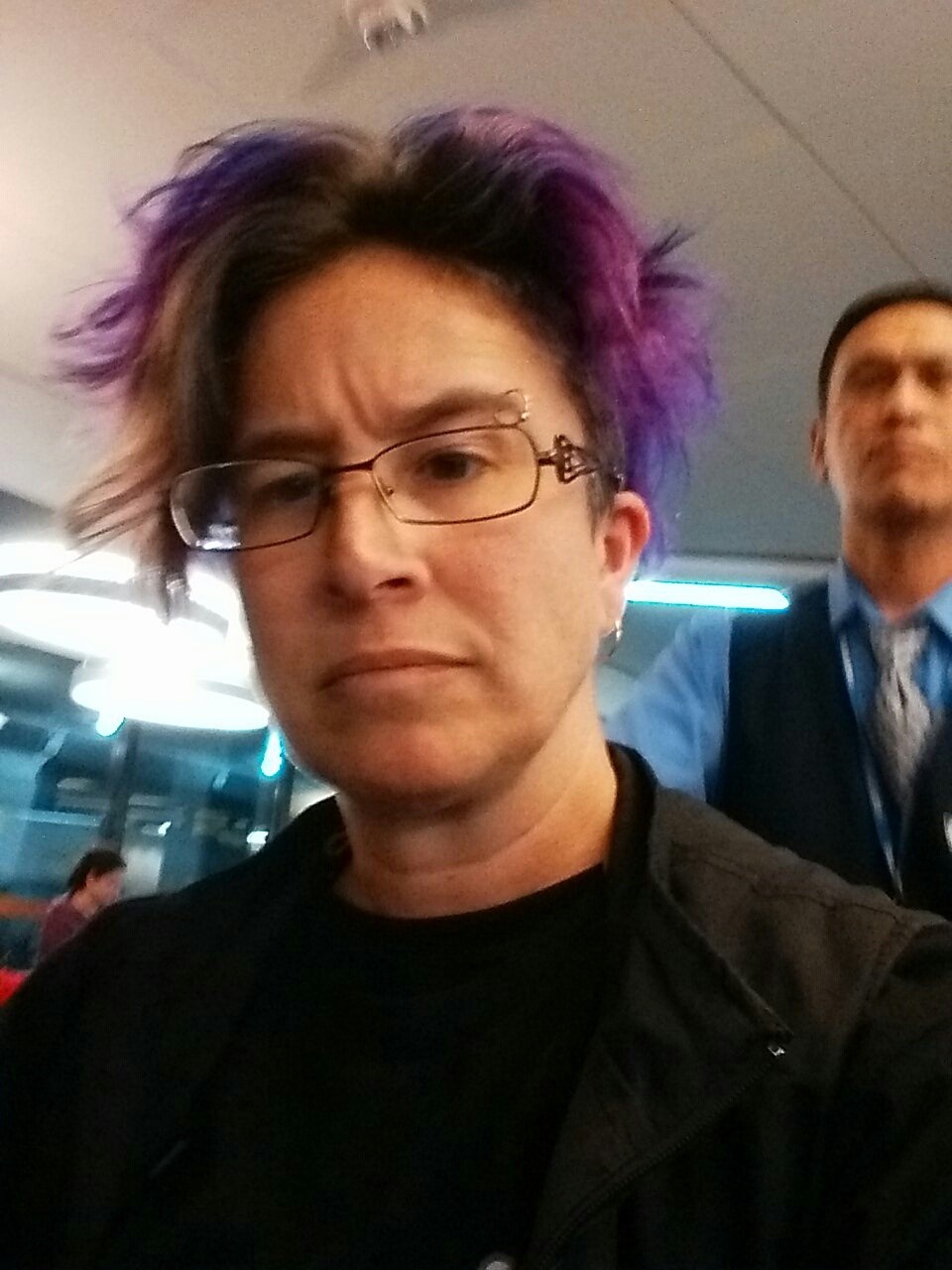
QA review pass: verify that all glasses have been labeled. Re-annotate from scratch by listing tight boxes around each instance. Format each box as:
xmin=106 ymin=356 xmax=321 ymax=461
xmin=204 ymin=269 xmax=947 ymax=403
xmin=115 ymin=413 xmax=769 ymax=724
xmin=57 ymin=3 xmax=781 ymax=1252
xmin=170 ymin=422 xmax=627 ymax=553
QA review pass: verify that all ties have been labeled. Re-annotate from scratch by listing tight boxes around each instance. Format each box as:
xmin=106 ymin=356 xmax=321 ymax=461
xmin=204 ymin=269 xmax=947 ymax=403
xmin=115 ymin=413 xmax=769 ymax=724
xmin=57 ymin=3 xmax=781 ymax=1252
xmin=868 ymin=623 xmax=932 ymax=820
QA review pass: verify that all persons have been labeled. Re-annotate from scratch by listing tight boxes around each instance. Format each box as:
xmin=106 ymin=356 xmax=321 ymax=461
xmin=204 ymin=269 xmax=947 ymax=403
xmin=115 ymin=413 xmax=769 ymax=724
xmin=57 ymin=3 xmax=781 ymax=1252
xmin=602 ymin=278 xmax=952 ymax=931
xmin=0 ymin=102 xmax=952 ymax=1270
